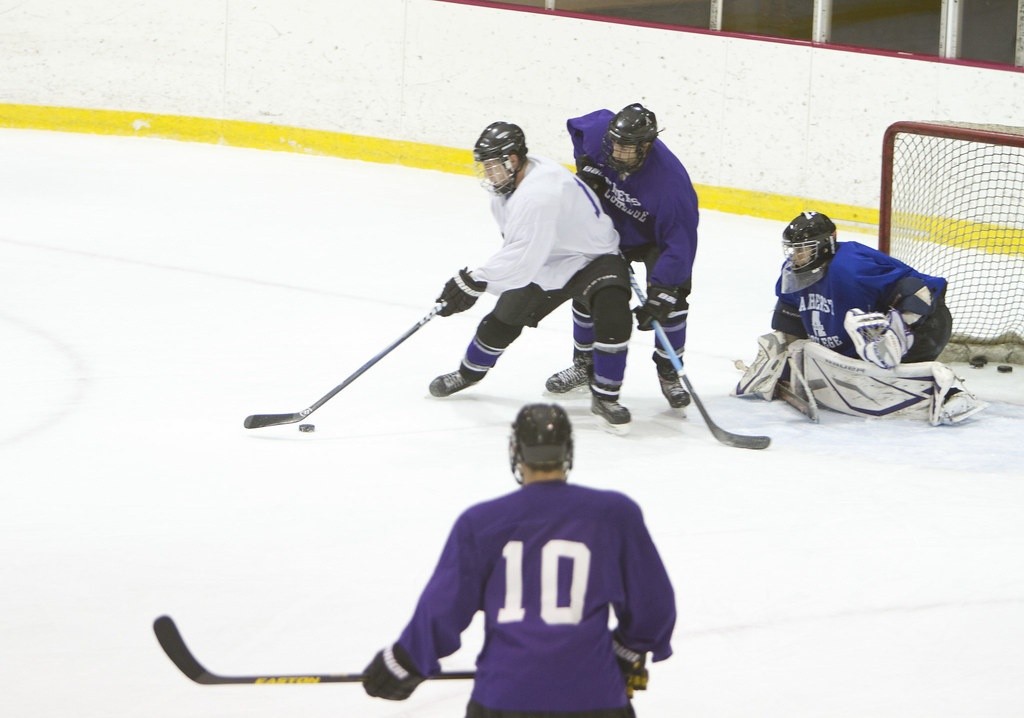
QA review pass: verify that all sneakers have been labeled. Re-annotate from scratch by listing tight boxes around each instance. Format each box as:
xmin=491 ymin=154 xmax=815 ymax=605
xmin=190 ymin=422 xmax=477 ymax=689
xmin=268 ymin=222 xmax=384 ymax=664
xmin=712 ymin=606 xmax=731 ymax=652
xmin=542 ymin=364 xmax=590 ymax=395
xmin=591 ymin=395 xmax=631 ymax=435
xmin=425 ymin=370 xmax=480 ymax=399
xmin=657 ymin=365 xmax=691 ymax=418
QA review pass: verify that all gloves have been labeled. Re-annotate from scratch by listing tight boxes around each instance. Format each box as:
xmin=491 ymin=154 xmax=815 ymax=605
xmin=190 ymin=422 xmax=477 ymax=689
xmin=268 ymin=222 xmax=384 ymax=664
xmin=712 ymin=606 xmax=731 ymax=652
xmin=636 ymin=286 xmax=679 ymax=330
xmin=362 ymin=642 xmax=428 ymax=701
xmin=435 ymin=266 xmax=488 ymax=317
xmin=613 ymin=628 xmax=641 ymax=685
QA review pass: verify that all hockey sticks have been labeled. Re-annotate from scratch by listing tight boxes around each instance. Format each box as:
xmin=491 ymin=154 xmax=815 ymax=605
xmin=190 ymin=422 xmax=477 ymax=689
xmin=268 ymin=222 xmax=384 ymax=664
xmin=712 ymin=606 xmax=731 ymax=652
xmin=152 ymin=612 xmax=651 ymax=693
xmin=618 ymin=247 xmax=774 ymax=452
xmin=241 ymin=298 xmax=449 ymax=432
xmin=734 ymin=353 xmax=822 ymax=426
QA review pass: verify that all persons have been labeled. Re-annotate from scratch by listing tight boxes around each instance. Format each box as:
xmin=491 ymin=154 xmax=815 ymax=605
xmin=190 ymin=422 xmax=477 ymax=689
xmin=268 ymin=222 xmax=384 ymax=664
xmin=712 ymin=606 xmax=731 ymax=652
xmin=429 ymin=120 xmax=632 ymax=426
xmin=729 ymin=210 xmax=1024 ymax=427
xmin=544 ymin=103 xmax=701 ymax=408
xmin=361 ymin=401 xmax=676 ymax=718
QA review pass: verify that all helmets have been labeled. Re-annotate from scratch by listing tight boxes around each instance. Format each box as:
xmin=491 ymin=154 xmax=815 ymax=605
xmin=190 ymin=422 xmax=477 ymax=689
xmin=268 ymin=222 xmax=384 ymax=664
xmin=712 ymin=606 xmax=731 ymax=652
xmin=509 ymin=404 xmax=573 ymax=484
xmin=473 ymin=122 xmax=528 ymax=196
xmin=601 ymin=103 xmax=659 ymax=175
xmin=782 ymin=211 xmax=837 ymax=294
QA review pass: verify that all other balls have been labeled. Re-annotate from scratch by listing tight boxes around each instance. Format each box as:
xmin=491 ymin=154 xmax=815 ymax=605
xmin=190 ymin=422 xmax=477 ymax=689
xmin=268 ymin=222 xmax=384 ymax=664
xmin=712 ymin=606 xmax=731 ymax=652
xmin=996 ymin=364 xmax=1013 ymax=372
xmin=969 ymin=359 xmax=986 ymax=369
xmin=296 ymin=423 xmax=316 ymax=432
